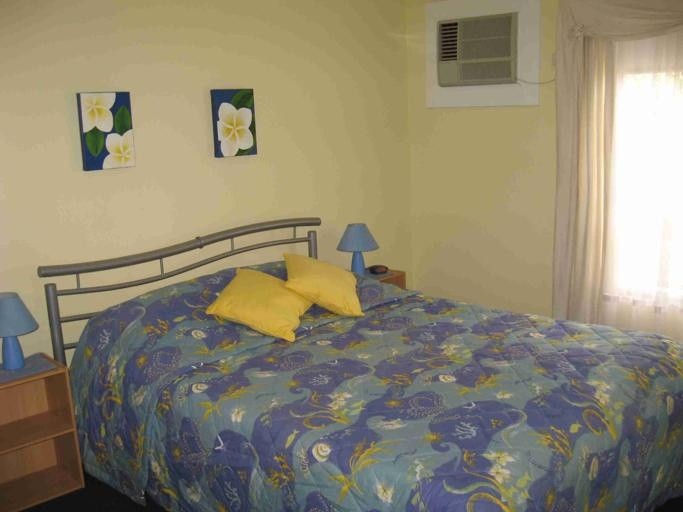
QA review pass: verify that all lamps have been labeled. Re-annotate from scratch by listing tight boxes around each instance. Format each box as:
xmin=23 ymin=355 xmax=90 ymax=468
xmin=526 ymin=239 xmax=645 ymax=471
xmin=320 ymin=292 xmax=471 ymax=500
xmin=336 ymin=221 xmax=379 ymax=280
xmin=1 ymin=293 xmax=40 ymax=370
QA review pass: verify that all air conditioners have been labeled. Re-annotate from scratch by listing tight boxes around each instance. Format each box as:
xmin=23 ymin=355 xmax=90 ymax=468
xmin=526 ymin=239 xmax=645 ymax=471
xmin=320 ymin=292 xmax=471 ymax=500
xmin=436 ymin=11 xmax=518 ymax=86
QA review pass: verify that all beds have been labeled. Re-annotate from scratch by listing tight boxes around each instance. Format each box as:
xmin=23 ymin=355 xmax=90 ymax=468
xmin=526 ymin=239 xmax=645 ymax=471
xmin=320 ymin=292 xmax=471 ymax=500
xmin=38 ymin=214 xmax=683 ymax=511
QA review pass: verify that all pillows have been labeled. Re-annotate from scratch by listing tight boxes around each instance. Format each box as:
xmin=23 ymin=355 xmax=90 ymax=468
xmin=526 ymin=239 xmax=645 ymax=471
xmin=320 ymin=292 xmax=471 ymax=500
xmin=205 ymin=250 xmax=368 ymax=344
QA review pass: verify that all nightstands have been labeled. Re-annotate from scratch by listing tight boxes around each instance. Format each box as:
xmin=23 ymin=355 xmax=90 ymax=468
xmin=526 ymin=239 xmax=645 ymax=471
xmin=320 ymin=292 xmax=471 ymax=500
xmin=368 ymin=268 xmax=407 ymax=292
xmin=0 ymin=353 xmax=87 ymax=512
